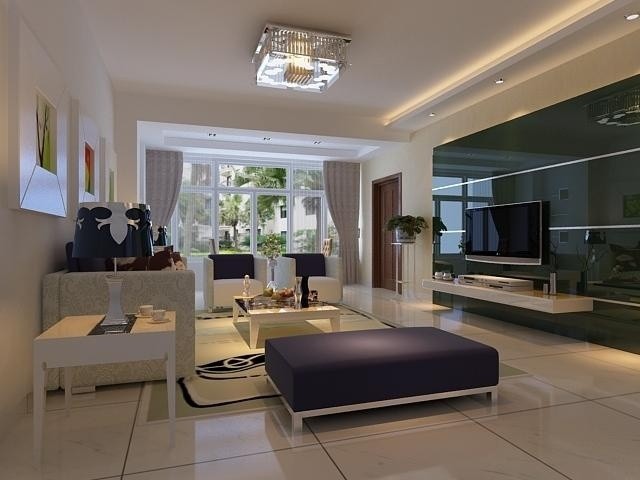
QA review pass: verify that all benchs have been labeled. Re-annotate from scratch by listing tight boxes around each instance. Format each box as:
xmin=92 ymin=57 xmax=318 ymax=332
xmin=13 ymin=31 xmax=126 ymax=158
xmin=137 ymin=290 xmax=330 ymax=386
xmin=264 ymin=327 xmax=499 ymax=433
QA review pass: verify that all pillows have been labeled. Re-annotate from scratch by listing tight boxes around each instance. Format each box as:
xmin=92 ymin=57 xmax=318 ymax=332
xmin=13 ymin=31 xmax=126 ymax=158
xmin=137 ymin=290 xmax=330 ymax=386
xmin=65 ymin=241 xmax=185 ymax=272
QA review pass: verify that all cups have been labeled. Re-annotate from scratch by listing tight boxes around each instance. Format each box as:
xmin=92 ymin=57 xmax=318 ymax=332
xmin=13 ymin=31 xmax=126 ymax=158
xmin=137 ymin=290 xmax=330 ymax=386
xmin=443 ymin=272 xmax=452 ymax=280
xmin=139 ymin=305 xmax=153 ymax=316
xmin=435 ymin=272 xmax=443 ymax=279
xmin=262 ymin=280 xmax=278 ymax=298
xmin=150 ymin=310 xmax=165 ymax=320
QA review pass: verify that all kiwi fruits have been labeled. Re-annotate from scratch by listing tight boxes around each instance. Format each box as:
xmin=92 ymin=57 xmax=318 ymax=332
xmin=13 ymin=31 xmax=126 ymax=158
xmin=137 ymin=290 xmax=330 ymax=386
xmin=279 ymin=288 xmax=293 ymax=297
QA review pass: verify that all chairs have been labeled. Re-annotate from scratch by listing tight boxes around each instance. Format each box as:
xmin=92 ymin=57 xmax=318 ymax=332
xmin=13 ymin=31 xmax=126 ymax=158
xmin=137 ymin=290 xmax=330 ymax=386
xmin=273 ymin=253 xmax=344 ymax=306
xmin=321 ymin=239 xmax=332 ymax=257
xmin=203 ymin=253 xmax=268 ymax=312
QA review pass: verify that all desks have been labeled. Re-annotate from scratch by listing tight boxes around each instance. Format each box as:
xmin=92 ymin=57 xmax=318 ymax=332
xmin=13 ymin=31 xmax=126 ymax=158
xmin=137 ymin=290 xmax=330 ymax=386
xmin=32 ymin=311 xmax=176 ymax=466
xmin=391 ymin=242 xmax=419 ymax=299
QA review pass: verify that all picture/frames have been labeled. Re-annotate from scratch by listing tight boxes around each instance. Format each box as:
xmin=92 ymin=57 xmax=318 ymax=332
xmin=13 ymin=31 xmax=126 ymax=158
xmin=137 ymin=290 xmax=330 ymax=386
xmin=6 ymin=14 xmax=118 ymax=220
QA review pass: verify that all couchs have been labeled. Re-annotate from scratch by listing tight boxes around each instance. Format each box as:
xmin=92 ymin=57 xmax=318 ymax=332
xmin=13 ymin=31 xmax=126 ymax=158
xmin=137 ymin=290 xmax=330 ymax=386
xmin=41 ymin=256 xmax=196 ymax=395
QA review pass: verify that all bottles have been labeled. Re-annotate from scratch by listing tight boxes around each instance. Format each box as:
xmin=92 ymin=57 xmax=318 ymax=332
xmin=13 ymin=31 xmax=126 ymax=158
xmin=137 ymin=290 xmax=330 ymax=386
xmin=243 ymin=274 xmax=251 ymax=301
xmin=294 ymin=277 xmax=302 ymax=309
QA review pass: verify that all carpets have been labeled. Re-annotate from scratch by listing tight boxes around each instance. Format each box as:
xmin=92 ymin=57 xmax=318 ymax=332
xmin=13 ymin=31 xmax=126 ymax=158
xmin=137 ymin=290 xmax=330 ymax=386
xmin=145 ymin=303 xmax=398 ymax=423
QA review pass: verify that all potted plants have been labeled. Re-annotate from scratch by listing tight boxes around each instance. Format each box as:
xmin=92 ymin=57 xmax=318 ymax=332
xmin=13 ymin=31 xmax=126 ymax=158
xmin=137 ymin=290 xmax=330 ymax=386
xmin=384 ymin=214 xmax=429 ymax=243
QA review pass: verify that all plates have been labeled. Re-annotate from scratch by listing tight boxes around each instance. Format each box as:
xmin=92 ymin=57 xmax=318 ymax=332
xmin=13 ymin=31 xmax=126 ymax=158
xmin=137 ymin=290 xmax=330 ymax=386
xmin=146 ymin=320 xmax=169 ymax=323
xmin=134 ymin=314 xmax=151 ymax=318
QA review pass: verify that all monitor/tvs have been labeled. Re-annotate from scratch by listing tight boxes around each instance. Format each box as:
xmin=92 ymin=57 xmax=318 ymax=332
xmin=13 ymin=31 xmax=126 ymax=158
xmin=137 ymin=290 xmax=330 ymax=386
xmin=463 ymin=200 xmax=543 ymax=266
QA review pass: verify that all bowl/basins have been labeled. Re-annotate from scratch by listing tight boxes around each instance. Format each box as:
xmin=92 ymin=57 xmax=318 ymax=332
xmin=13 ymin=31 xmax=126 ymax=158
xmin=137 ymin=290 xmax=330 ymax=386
xmin=273 ymin=295 xmax=293 ymax=302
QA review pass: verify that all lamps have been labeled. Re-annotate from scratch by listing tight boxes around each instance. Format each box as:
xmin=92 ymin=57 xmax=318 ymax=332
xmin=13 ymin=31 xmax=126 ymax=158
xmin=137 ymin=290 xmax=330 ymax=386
xmin=72 ymin=201 xmax=154 ymax=326
xmin=584 ymin=231 xmax=606 ymax=262
xmin=250 ymin=21 xmax=353 ymax=94
xmin=152 ymin=225 xmax=166 ymax=247
xmin=583 ymin=85 xmax=640 ymax=129
